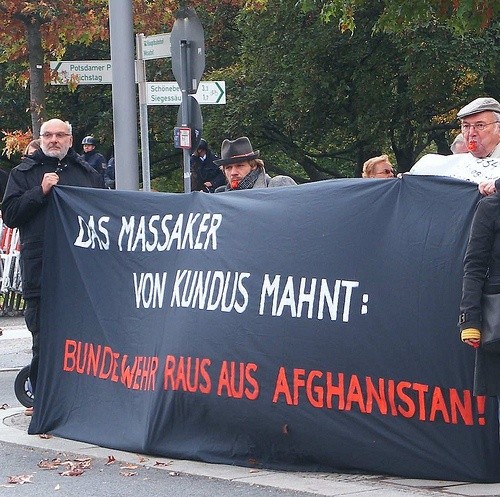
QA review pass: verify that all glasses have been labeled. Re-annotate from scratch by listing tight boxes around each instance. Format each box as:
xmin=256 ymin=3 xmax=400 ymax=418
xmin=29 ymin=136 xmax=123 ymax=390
xmin=376 ymin=170 xmax=393 ymax=175
xmin=38 ymin=132 xmax=71 ymax=138
xmin=458 ymin=121 xmax=499 ymax=131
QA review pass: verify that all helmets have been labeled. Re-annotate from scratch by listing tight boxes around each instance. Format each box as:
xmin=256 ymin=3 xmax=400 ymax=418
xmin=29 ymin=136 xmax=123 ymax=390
xmin=81 ymin=136 xmax=95 ymax=145
xmin=197 ymin=139 xmax=209 ymax=149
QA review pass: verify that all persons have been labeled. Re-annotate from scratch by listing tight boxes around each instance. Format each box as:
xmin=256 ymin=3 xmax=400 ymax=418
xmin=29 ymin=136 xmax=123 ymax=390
xmin=190 ymin=138 xmax=226 ymax=193
xmin=451 ymin=97 xmax=500 ymax=196
xmin=457 ymin=179 xmax=500 ymax=398
xmin=1 ymin=118 xmax=107 ymax=416
xmin=213 ymin=137 xmax=297 ymax=193
xmin=25 ymin=134 xmax=115 ymax=190
xmin=362 ymin=155 xmax=395 ymax=179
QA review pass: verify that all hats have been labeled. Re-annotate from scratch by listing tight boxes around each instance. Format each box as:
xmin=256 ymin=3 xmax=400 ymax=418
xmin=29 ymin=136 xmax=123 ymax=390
xmin=457 ymin=96 xmax=500 ymax=119
xmin=213 ymin=137 xmax=260 ymax=165
